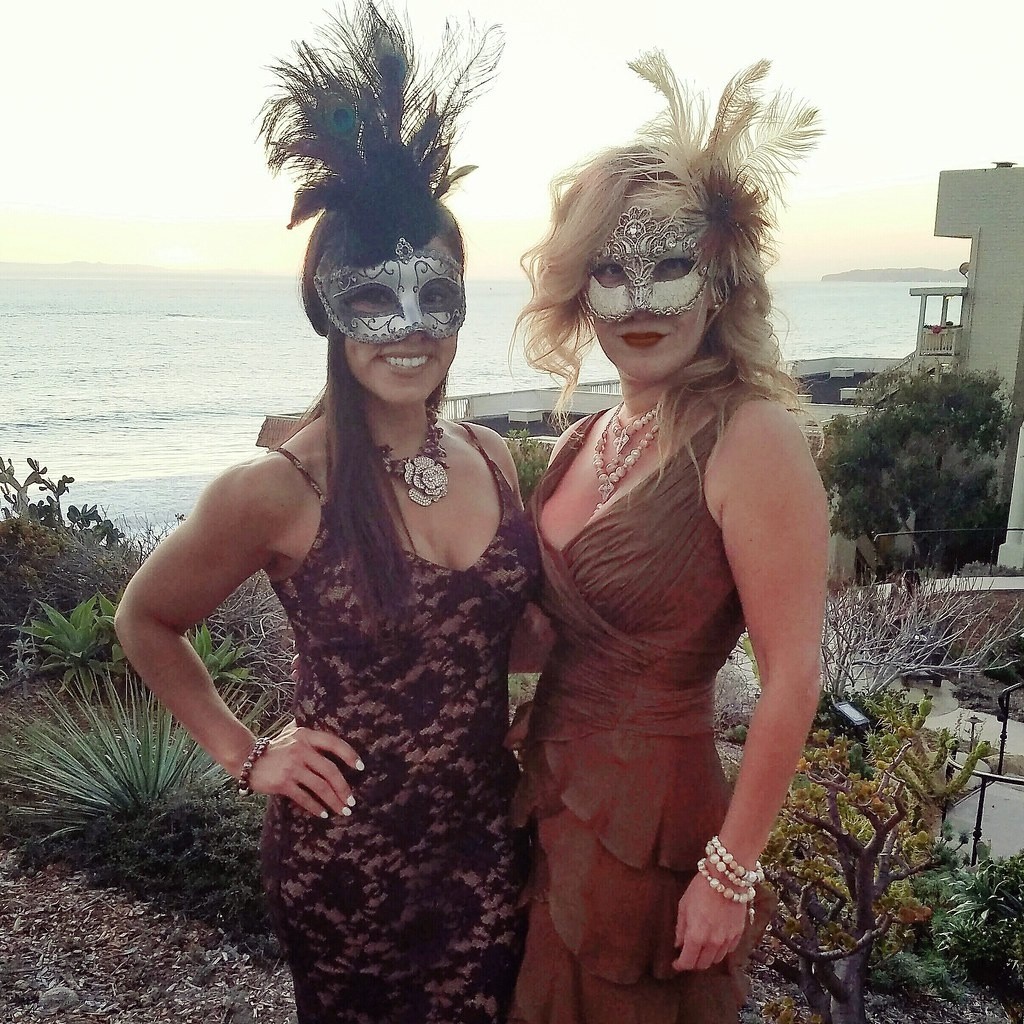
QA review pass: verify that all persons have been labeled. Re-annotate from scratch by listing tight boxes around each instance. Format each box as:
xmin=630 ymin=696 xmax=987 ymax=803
xmin=113 ymin=3 xmax=538 ymax=1024
xmin=289 ymin=54 xmax=832 ymax=1023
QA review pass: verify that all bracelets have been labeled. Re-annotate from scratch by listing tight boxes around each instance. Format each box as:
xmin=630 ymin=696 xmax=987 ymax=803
xmin=697 ymin=837 xmax=765 ymax=904
xmin=238 ymin=738 xmax=270 ymax=797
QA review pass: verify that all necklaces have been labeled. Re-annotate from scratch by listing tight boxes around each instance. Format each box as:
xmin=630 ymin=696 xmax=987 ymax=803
xmin=594 ymin=401 xmax=658 ymax=517
xmin=377 ymin=407 xmax=451 ymax=507
